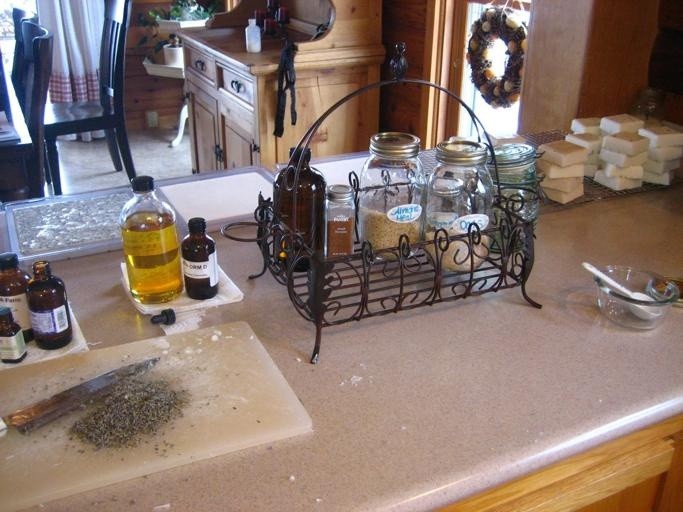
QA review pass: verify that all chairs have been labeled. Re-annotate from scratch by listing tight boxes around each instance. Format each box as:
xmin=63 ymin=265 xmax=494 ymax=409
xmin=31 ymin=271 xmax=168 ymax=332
xmin=11 ymin=7 xmax=53 ymax=197
xmin=44 ymin=18 xmax=137 ymax=194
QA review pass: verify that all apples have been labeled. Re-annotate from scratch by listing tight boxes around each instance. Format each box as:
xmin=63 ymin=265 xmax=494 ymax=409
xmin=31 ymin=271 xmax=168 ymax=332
xmin=170 ymin=8 xmax=181 ymax=16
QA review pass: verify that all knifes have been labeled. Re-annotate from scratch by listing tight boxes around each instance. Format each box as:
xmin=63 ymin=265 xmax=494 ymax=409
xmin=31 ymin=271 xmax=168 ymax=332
xmin=5 ymin=355 xmax=163 ymax=435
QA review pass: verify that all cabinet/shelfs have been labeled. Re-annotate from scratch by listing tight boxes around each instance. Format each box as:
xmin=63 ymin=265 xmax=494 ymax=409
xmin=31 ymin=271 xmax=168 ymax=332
xmin=179 ymin=1 xmax=387 ymax=176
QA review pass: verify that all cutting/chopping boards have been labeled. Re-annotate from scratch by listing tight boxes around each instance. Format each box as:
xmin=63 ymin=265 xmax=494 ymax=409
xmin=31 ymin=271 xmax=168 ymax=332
xmin=2 ymin=322 xmax=311 ymax=512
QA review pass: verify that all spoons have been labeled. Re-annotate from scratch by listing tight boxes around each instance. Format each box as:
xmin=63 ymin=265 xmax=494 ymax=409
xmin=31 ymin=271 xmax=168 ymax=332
xmin=580 ymin=261 xmax=662 ymax=320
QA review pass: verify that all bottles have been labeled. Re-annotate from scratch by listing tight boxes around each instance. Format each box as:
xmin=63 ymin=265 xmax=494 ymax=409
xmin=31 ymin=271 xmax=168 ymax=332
xmin=115 ymin=176 xmax=183 ymax=306
xmin=266 ymin=130 xmax=540 ymax=275
xmin=0 ymin=250 xmax=72 ymax=364
xmin=179 ymin=218 xmax=220 ymax=300
xmin=245 ymin=18 xmax=263 ymax=54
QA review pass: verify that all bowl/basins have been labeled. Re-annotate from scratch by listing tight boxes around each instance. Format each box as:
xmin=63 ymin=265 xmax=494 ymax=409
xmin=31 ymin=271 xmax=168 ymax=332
xmin=593 ymin=264 xmax=681 ymax=330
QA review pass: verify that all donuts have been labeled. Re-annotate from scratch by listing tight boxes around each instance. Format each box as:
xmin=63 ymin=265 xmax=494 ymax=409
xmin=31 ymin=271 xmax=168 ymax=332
xmin=466 ymin=8 xmax=529 ymax=109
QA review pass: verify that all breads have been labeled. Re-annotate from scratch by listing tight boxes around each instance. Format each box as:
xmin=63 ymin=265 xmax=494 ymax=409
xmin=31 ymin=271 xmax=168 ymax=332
xmin=537 ymin=113 xmax=683 ymax=204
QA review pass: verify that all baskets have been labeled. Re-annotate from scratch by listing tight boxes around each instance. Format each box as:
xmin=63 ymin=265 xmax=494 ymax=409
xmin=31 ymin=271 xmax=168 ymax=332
xmin=221 ymin=80 xmax=548 ymax=364
